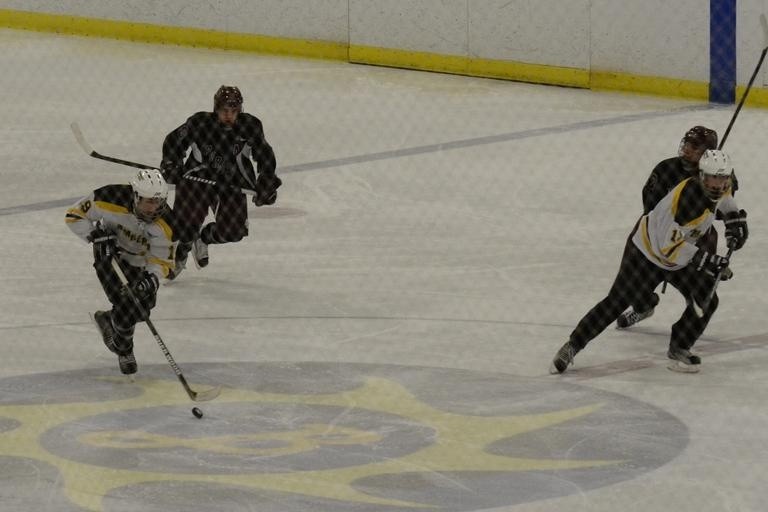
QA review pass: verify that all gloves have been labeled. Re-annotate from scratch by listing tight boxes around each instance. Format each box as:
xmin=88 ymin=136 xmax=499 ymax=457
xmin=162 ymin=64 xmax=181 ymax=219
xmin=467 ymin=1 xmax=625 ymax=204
xmin=692 ymin=248 xmax=733 ymax=281
xmin=160 ymin=160 xmax=186 ymax=184
xmin=724 ymin=208 xmax=748 ymax=250
xmin=86 ymin=228 xmax=117 ymax=265
xmin=253 ymin=174 xmax=281 ymax=206
xmin=120 ymin=271 xmax=159 ymax=302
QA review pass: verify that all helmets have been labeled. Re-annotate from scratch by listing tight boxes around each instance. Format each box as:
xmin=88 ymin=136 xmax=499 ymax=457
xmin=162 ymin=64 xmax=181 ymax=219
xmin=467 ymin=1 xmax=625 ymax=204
xmin=685 ymin=126 xmax=732 ymax=182
xmin=214 ymin=84 xmax=243 ymax=112
xmin=128 ymin=169 xmax=168 ymax=213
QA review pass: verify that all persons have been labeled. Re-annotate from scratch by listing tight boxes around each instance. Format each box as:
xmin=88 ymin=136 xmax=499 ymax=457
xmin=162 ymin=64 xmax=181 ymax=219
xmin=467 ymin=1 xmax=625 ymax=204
xmin=159 ymin=85 xmax=282 ymax=280
xmin=551 ymin=149 xmax=748 ymax=374
xmin=64 ymin=169 xmax=181 ymax=374
xmin=615 ymin=127 xmax=739 ymax=328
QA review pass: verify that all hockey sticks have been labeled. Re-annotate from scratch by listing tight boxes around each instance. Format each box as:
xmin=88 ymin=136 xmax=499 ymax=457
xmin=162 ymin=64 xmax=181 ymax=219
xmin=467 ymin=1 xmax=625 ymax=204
xmin=71 ymin=121 xmax=258 ymax=197
xmin=693 ymin=238 xmax=739 ymax=318
xmin=110 ymin=257 xmax=221 ymax=402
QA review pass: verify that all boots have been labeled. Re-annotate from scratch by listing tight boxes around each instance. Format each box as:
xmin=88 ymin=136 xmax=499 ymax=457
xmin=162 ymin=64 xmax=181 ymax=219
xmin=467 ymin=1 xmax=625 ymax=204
xmin=553 ymin=341 xmax=579 ymax=372
xmin=617 ymin=307 xmax=654 ymax=327
xmin=94 ymin=310 xmax=137 ymax=374
xmin=166 ymin=252 xmax=188 ymax=280
xmin=667 ymin=344 xmax=701 ymax=365
xmin=194 ymin=237 xmax=209 ymax=268
